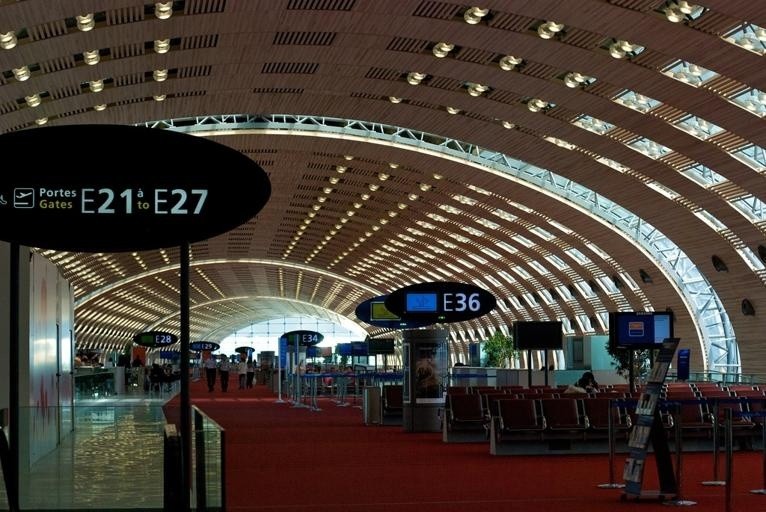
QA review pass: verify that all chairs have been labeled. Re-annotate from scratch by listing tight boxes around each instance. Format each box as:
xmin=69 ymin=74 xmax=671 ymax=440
xmin=381 ymin=378 xmax=766 ymax=457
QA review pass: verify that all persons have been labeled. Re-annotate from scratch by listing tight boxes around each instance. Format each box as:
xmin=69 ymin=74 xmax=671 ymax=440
xmin=246 ymin=360 xmax=255 ymax=388
xmin=75 ymin=353 xmax=172 ymax=392
xmin=237 ymin=357 xmax=247 ymax=390
xmin=305 ymin=363 xmax=355 ymax=386
xmin=203 ymin=353 xmax=216 ymax=392
xmin=575 ymin=372 xmax=599 ymax=390
xmin=220 ymin=354 xmax=230 ymax=392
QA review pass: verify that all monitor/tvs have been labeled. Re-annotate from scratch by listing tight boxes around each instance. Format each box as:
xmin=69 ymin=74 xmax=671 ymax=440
xmin=512 ymin=320 xmax=564 ymax=352
xmin=654 ymin=309 xmax=675 ymax=346
xmin=368 ymin=300 xmax=399 ymax=320
xmin=405 ymin=292 xmax=438 ymax=312
xmin=608 ymin=311 xmax=655 ymax=349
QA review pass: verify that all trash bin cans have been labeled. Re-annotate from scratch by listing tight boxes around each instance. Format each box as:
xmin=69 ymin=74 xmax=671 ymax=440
xmin=364 ymin=386 xmax=381 ymax=426
xmin=273 ymin=368 xmax=286 ymax=394
xmin=115 ymin=366 xmax=126 ymax=394
